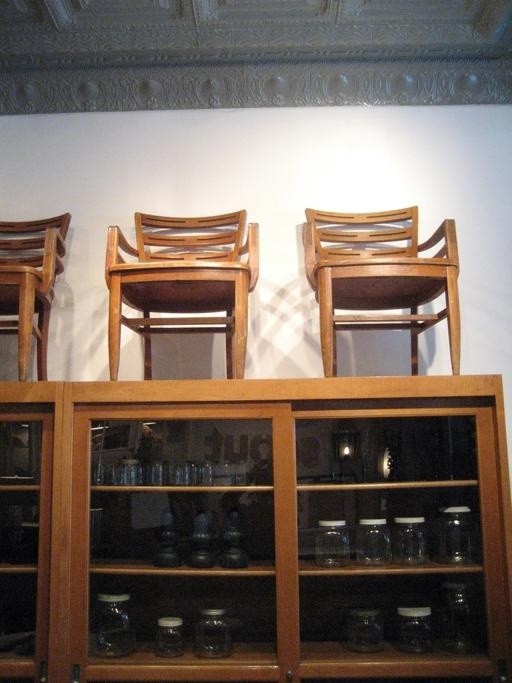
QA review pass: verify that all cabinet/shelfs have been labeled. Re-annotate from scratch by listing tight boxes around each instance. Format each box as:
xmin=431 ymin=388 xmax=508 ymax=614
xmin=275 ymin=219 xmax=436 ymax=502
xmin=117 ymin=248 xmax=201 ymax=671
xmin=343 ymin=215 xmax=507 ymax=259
xmin=0 ymin=374 xmax=512 ymax=683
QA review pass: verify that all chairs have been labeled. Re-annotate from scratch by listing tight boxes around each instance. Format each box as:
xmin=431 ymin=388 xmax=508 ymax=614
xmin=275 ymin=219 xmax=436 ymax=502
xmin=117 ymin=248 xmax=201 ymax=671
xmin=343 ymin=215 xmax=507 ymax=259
xmin=0 ymin=211 xmax=71 ymax=381
xmin=300 ymin=205 xmax=463 ymax=377
xmin=105 ymin=208 xmax=260 ymax=379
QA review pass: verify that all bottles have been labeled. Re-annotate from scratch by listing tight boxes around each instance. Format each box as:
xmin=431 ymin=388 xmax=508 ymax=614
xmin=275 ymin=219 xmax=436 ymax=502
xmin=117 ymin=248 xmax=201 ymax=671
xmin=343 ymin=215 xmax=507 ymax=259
xmin=91 ymin=454 xmax=251 ymax=659
xmin=315 ymin=506 xmax=480 ymax=654
